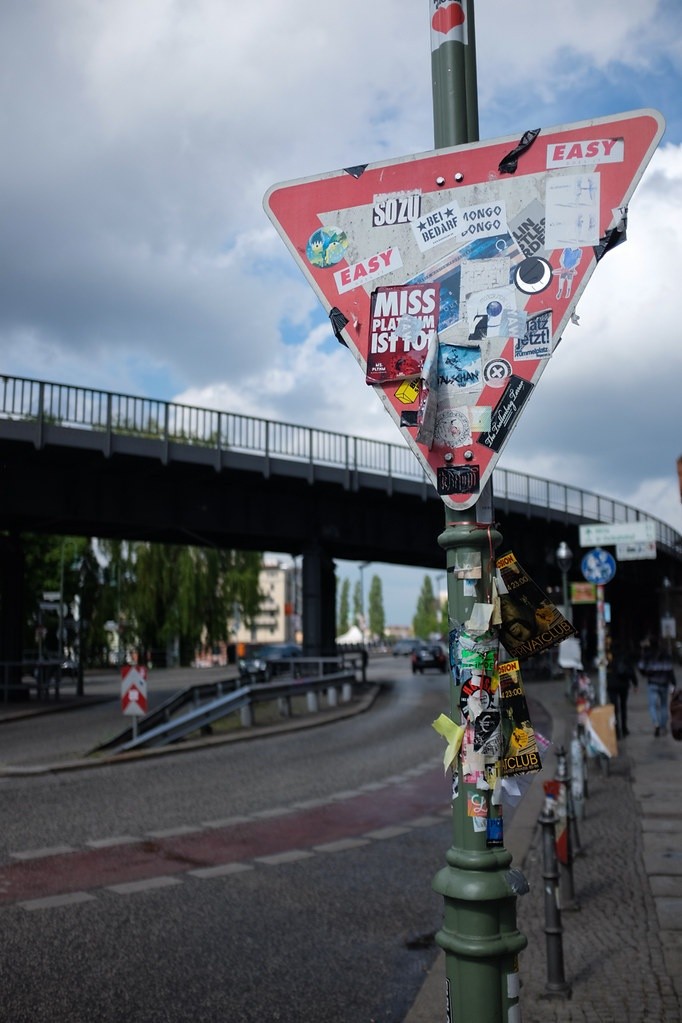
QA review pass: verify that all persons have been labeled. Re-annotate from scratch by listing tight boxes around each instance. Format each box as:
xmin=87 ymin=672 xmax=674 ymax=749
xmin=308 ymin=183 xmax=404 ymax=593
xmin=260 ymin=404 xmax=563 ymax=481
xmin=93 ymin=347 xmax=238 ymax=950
xmin=638 ymin=641 xmax=677 ymax=737
xmin=607 ymin=643 xmax=639 ymax=741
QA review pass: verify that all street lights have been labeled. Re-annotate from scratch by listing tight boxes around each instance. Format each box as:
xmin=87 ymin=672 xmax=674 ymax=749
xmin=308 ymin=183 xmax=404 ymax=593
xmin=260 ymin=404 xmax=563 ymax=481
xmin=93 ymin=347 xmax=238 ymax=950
xmin=555 ymin=542 xmax=573 ymax=694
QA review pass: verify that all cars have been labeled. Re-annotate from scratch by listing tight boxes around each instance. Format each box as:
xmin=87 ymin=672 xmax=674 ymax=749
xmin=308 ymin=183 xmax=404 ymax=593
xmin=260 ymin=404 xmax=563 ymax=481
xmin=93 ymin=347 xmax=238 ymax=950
xmin=235 ymin=642 xmax=301 ymax=681
xmin=411 ymin=644 xmax=445 ymax=674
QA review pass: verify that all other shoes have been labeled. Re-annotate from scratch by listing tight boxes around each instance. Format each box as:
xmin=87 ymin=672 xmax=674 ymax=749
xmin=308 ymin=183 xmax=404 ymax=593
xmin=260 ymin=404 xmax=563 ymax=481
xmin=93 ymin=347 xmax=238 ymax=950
xmin=622 ymin=728 xmax=629 ymax=737
xmin=660 ymin=728 xmax=666 ymax=736
xmin=655 ymin=724 xmax=660 ymax=737
xmin=616 ymin=728 xmax=621 ymax=740
